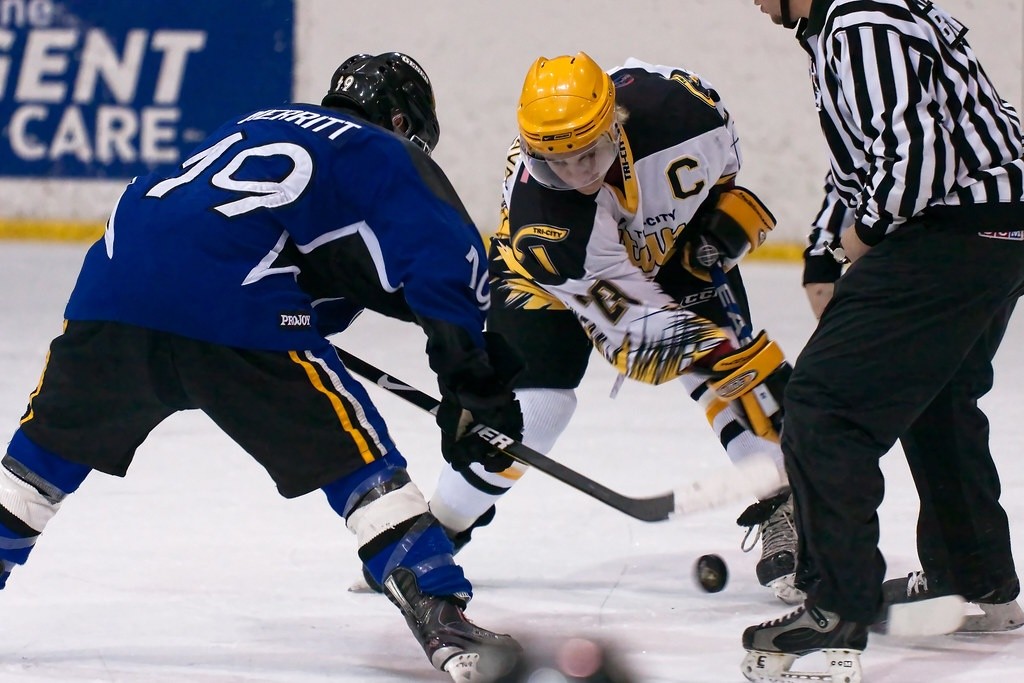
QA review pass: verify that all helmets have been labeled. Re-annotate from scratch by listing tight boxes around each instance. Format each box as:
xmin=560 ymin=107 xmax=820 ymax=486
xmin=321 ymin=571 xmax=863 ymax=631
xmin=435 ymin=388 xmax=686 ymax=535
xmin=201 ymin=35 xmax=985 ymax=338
xmin=516 ymin=51 xmax=620 ymax=191
xmin=320 ymin=51 xmax=440 ymax=158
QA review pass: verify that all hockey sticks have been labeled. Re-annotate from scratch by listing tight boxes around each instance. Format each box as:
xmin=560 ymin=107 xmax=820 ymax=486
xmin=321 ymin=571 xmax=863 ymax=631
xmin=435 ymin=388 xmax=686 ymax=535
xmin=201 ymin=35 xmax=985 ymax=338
xmin=701 ymin=235 xmax=968 ymax=637
xmin=336 ymin=348 xmax=782 ymax=525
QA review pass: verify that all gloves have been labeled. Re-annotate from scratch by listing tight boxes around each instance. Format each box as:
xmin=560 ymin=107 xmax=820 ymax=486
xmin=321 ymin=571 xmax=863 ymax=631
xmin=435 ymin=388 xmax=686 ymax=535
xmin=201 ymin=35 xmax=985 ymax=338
xmin=673 ymin=185 xmax=776 ymax=294
xmin=441 ymin=395 xmax=528 ymax=496
xmin=688 ymin=330 xmax=793 ymax=445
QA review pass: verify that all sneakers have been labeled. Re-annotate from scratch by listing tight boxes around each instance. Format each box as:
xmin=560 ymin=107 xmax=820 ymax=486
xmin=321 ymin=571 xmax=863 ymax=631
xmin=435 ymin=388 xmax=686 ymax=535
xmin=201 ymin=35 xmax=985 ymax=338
xmin=743 ymin=581 xmax=869 ymax=683
xmin=866 ymin=570 xmax=1024 ymax=661
xmin=380 ymin=565 xmax=525 ymax=683
xmin=347 ymin=502 xmax=497 ymax=594
xmin=737 ymin=486 xmax=808 ymax=605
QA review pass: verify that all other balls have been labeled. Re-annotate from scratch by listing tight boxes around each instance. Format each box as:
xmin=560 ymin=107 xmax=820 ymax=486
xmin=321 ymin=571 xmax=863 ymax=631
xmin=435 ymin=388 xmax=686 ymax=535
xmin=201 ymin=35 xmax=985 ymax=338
xmin=694 ymin=552 xmax=727 ymax=594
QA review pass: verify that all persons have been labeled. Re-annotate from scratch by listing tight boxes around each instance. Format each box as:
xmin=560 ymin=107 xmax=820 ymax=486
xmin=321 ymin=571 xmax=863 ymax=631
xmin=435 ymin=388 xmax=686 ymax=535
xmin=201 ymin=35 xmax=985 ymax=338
xmin=0 ymin=50 xmax=528 ymax=683
xmin=740 ymin=0 xmax=1024 ymax=683
xmin=345 ymin=49 xmax=811 ymax=607
